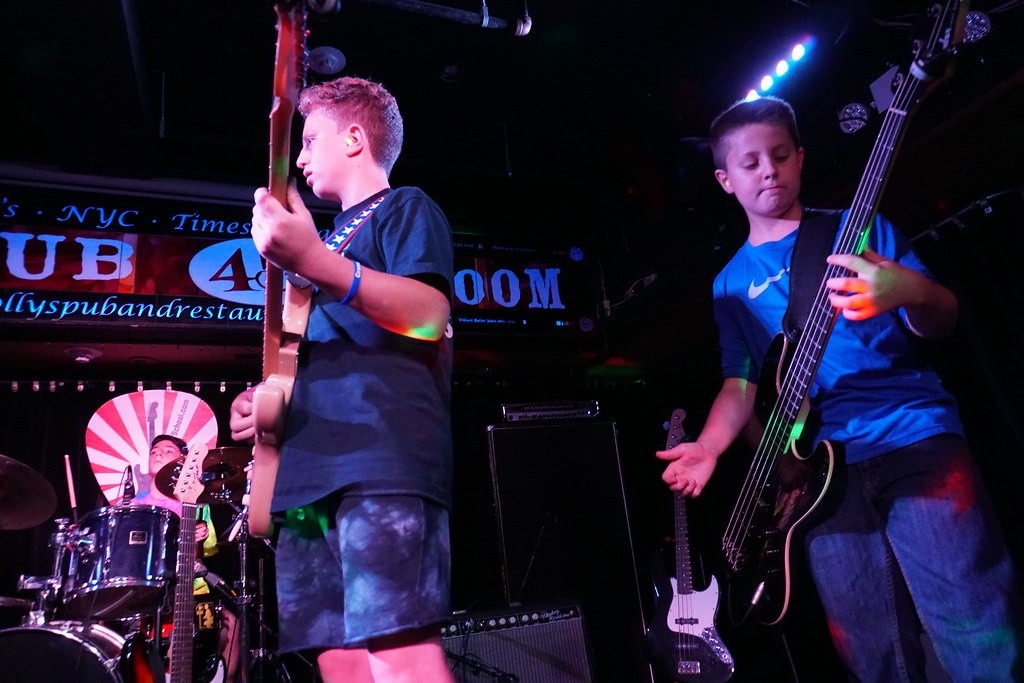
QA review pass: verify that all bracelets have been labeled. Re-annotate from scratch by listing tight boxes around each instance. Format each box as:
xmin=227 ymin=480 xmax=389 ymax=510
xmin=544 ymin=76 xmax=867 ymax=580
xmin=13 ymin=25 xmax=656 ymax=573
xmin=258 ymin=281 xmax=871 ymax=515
xmin=338 ymin=260 xmax=361 ymax=306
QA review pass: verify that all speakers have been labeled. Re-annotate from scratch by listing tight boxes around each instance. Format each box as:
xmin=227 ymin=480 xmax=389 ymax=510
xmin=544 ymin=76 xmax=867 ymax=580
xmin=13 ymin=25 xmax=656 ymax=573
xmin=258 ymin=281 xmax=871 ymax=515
xmin=485 ymin=416 xmax=654 ymax=683
xmin=438 ymin=619 xmax=592 ymax=683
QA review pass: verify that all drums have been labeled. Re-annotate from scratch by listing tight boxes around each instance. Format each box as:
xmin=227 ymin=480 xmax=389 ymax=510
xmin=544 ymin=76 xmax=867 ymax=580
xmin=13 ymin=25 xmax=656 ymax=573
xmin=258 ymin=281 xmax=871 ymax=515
xmin=0 ymin=620 xmax=129 ymax=683
xmin=62 ymin=505 xmax=181 ymax=621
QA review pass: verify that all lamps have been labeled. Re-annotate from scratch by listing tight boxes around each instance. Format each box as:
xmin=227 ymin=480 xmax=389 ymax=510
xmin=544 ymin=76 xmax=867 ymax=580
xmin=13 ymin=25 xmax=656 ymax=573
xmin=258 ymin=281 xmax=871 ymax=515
xmin=833 ymin=12 xmax=991 ymax=138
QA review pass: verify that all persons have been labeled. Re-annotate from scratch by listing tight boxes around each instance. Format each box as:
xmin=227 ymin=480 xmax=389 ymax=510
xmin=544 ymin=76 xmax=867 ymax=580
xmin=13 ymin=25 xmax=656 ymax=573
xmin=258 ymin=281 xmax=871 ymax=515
xmin=230 ymin=76 xmax=454 ymax=683
xmin=656 ymin=98 xmax=1024 ymax=683
xmin=66 ymin=435 xmax=239 ymax=683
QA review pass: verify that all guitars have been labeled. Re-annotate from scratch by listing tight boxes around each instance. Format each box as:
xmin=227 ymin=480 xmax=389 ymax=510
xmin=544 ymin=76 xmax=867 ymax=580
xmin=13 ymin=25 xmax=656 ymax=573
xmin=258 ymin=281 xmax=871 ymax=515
xmin=697 ymin=0 xmax=990 ymax=627
xmin=115 ymin=442 xmax=227 ymax=683
xmin=650 ymin=407 xmax=738 ymax=683
xmin=240 ymin=0 xmax=341 ymax=539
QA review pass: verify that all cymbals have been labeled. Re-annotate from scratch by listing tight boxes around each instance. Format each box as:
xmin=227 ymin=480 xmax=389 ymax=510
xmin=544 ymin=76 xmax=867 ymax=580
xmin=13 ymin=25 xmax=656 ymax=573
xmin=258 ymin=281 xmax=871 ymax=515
xmin=1 ymin=454 xmax=58 ymax=530
xmin=154 ymin=446 xmax=254 ymax=506
